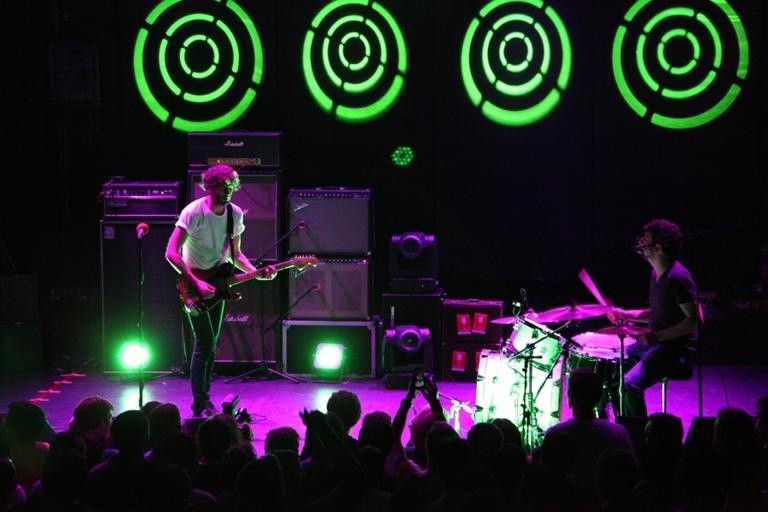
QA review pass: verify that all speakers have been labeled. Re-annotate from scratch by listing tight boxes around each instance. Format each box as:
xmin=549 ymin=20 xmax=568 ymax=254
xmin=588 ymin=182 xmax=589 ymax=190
xmin=213 ymin=260 xmax=277 ymax=363
xmin=100 ymin=218 xmax=188 ymax=375
xmin=381 ymin=291 xmax=448 ymax=388
xmin=288 ymin=187 xmax=373 ymax=257
xmin=286 ymin=256 xmax=370 ymax=321
xmin=102 ymin=180 xmax=180 ymax=219
xmin=386 ymin=232 xmax=441 ymax=293
xmin=281 ymin=319 xmax=378 ymax=383
xmin=0 ymin=321 xmax=42 ymax=371
xmin=186 ymin=161 xmax=277 ymax=261
xmin=685 ymin=416 xmax=715 ymax=448
xmin=188 ymin=131 xmax=281 ymax=176
xmin=0 ymin=273 xmax=41 ymax=323
xmin=442 ymin=341 xmax=504 ymax=382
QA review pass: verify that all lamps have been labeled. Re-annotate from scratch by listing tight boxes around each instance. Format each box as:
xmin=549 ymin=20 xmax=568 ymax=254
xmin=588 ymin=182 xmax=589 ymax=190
xmin=310 ymin=342 xmax=344 ymax=384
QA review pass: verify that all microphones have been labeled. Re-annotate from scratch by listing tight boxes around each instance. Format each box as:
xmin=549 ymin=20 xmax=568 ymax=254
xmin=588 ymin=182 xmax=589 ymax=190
xmin=520 ymin=288 xmax=529 ymax=310
xmin=631 ymin=246 xmax=643 ymax=254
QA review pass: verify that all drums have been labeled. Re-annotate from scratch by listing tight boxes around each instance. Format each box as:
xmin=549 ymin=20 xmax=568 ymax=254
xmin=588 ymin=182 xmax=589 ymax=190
xmin=502 ymin=318 xmax=568 ymax=373
xmin=568 ymin=333 xmax=638 ymax=383
xmin=474 ymin=349 xmax=565 ymax=456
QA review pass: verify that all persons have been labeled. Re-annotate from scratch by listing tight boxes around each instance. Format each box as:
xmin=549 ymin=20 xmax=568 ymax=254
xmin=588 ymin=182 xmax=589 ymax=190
xmin=162 ymin=166 xmax=279 ymax=426
xmin=625 ymin=220 xmax=704 ymax=430
xmin=2 ymin=367 xmax=768 ymax=510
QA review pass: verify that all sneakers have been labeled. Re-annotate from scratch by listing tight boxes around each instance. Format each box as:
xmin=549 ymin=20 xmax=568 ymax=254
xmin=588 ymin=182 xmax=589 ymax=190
xmin=191 ymin=407 xmax=216 ymax=420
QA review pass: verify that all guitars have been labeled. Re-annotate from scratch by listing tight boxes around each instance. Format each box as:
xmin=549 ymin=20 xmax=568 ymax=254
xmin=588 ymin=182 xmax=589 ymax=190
xmin=176 ymin=255 xmax=318 ymax=317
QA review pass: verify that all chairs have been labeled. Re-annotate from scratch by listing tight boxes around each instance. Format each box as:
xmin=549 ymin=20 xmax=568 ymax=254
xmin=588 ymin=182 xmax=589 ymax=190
xmin=628 ymin=287 xmax=702 ymax=418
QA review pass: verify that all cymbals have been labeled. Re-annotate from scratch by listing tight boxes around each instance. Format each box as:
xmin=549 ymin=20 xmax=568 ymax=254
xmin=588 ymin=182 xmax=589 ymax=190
xmin=596 ymin=325 xmax=652 ymax=335
xmin=490 ymin=305 xmax=613 ymax=325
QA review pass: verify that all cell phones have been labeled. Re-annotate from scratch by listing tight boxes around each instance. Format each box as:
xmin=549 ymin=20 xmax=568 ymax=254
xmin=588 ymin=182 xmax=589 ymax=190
xmin=415 ymin=366 xmax=426 ymax=389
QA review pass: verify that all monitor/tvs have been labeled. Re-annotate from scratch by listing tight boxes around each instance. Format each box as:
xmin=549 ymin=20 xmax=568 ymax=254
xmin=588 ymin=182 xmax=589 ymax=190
xmin=442 ymin=298 xmax=504 ymax=346
